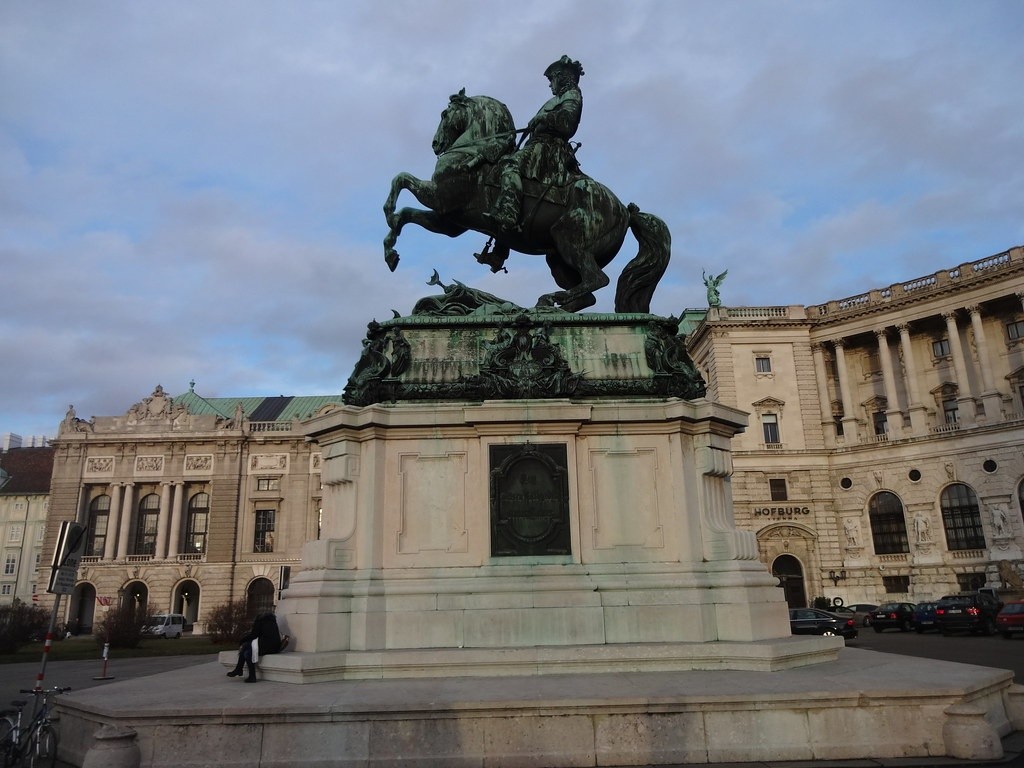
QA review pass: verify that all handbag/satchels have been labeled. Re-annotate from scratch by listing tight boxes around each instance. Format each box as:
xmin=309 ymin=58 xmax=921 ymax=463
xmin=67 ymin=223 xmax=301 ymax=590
xmin=281 ymin=635 xmax=289 ymax=651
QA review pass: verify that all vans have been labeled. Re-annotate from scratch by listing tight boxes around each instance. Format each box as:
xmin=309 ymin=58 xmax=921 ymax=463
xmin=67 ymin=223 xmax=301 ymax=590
xmin=139 ymin=614 xmax=183 ymax=639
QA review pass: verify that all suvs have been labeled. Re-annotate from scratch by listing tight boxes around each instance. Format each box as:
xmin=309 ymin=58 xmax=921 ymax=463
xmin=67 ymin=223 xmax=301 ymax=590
xmin=935 ymin=592 xmax=1004 ymax=637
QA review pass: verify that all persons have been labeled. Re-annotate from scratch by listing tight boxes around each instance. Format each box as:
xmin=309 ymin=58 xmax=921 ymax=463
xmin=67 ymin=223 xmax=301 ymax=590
xmin=986 ymin=504 xmax=1007 ymax=534
xmin=474 ymin=58 xmax=584 ymax=274
xmin=914 ymin=511 xmax=930 ymax=540
xmin=65 ymin=405 xmax=75 ymax=430
xmin=845 ymin=519 xmax=858 ymax=544
xmin=235 ymin=401 xmax=243 ymax=427
xmin=226 ymin=613 xmax=282 ymax=682
xmin=701 ymin=271 xmax=719 ymax=304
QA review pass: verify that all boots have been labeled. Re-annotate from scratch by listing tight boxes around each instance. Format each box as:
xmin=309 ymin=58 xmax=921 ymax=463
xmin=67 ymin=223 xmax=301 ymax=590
xmin=227 ymin=654 xmax=245 ymax=676
xmin=245 ymin=656 xmax=257 ymax=683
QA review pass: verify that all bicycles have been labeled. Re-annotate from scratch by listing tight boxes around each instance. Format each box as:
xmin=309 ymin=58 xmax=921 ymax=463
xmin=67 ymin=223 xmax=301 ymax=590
xmin=0 ymin=686 xmax=72 ymax=768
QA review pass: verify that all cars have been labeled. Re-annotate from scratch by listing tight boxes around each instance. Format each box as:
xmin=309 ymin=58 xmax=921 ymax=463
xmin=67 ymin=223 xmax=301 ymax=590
xmin=872 ymin=601 xmax=938 ymax=633
xmin=995 ymin=600 xmax=1024 ymax=639
xmin=789 ymin=608 xmax=859 ymax=639
xmin=831 ymin=603 xmax=878 ymax=627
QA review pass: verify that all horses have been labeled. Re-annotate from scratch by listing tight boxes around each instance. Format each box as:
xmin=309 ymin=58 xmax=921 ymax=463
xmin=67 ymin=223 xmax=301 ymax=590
xmin=384 ymin=87 xmax=672 ymax=313
